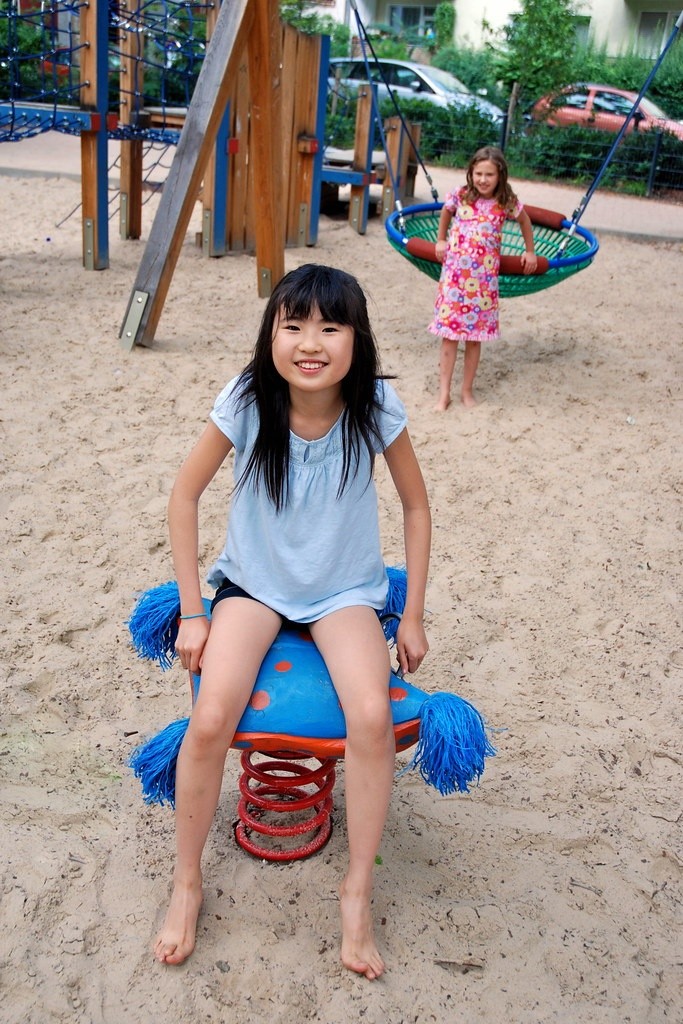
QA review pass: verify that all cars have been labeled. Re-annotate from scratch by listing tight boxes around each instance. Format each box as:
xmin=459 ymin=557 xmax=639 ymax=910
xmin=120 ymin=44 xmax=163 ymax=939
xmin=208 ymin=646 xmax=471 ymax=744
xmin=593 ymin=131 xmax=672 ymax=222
xmin=327 ymin=54 xmax=507 ymax=125
xmin=39 ymin=44 xmax=120 ymax=91
xmin=531 ymin=84 xmax=683 ymax=141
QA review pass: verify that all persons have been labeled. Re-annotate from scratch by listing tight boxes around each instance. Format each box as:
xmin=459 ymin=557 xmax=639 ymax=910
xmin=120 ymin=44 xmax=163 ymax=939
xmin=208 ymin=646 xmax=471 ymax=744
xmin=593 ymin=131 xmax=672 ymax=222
xmin=435 ymin=145 xmax=538 ymax=411
xmin=151 ymin=264 xmax=434 ymax=978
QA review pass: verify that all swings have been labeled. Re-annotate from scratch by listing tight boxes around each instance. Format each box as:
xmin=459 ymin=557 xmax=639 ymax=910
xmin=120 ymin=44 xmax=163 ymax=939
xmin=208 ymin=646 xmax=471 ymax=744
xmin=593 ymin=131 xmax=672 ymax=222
xmin=347 ymin=1 xmax=683 ymax=301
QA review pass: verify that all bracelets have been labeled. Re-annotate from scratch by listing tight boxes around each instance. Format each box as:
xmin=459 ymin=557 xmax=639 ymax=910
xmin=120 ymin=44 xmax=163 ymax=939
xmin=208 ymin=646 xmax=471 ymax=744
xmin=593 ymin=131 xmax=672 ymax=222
xmin=180 ymin=612 xmax=207 ymax=620
